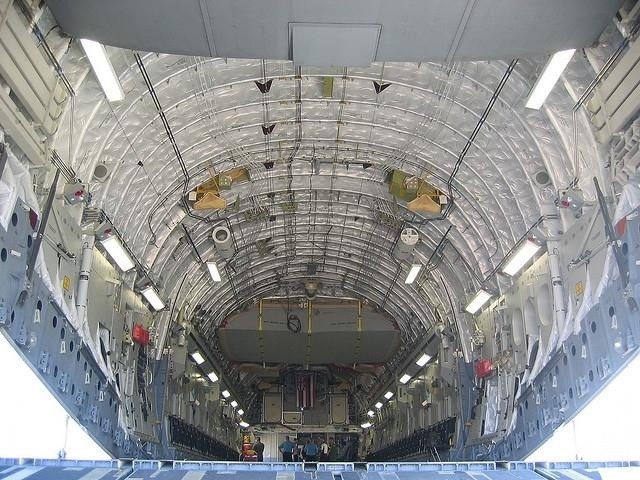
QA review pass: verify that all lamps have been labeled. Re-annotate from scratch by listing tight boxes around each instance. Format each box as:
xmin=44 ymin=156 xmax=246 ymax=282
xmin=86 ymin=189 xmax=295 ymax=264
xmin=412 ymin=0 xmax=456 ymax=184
xmin=79 ymin=39 xmax=125 ymax=102
xmin=102 ymin=236 xmax=251 ymax=431
xmin=361 ymin=236 xmax=542 ymax=428
xmin=523 ymin=49 xmax=578 ymax=110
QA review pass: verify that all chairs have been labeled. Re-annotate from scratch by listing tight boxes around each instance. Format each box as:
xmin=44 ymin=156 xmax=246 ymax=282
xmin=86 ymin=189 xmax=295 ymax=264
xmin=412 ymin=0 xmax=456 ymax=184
xmin=364 ymin=416 xmax=455 ymax=463
xmin=170 ymin=416 xmax=239 ymax=461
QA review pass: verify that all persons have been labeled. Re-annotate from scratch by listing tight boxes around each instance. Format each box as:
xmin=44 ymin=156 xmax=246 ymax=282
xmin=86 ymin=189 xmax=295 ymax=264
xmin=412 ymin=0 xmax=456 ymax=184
xmin=252 ymin=437 xmax=264 ymax=462
xmin=278 ymin=435 xmax=296 ymax=462
xmin=292 ymin=437 xmax=331 ymax=462
